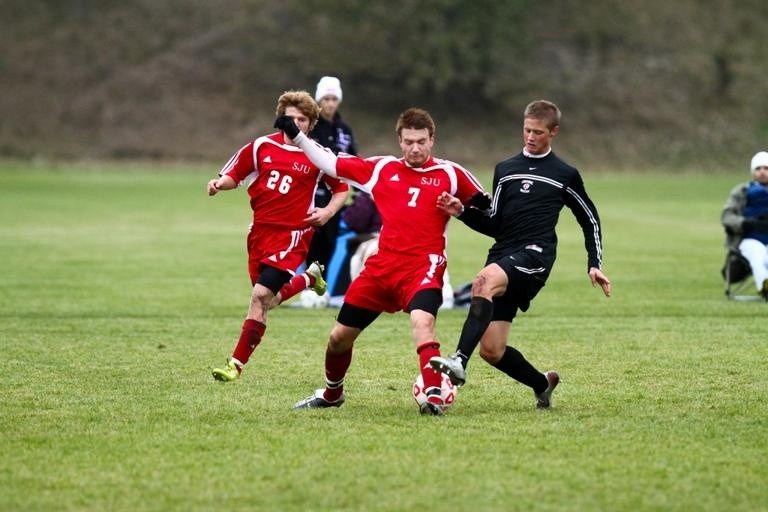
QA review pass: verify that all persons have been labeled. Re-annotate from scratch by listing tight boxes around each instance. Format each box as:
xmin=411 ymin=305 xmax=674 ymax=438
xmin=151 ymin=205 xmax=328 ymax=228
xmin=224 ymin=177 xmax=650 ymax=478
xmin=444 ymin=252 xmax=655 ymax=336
xmin=429 ymin=97 xmax=612 ymax=406
xmin=273 ymin=105 xmax=494 ymax=417
xmin=207 ymin=86 xmax=328 ymax=382
xmin=720 ymin=151 xmax=768 ymax=301
xmin=298 ymin=75 xmax=359 ymax=308
xmin=331 ymin=192 xmax=383 ymax=297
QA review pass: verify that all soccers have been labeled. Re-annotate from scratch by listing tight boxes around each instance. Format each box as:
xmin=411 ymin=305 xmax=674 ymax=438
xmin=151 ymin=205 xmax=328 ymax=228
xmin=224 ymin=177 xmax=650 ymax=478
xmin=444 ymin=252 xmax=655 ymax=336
xmin=412 ymin=371 xmax=455 ymax=411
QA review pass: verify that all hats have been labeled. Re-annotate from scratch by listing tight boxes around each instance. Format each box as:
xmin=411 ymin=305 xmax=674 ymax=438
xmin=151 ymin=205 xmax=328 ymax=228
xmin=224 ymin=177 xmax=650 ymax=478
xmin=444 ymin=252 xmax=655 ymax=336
xmin=314 ymin=76 xmax=343 ymax=104
xmin=750 ymin=151 xmax=768 ymax=176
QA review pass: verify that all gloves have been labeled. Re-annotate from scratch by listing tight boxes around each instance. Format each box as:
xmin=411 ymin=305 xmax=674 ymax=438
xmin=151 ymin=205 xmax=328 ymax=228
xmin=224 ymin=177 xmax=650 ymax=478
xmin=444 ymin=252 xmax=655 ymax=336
xmin=274 ymin=115 xmax=300 ymax=140
xmin=468 ymin=192 xmax=492 ymax=210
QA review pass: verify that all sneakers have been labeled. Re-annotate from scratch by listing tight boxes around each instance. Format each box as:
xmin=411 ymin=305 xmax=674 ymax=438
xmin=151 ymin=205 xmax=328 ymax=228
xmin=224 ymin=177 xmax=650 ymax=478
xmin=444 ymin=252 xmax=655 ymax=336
xmin=536 ymin=371 xmax=559 ymax=408
xmin=420 ymin=402 xmax=445 ymax=416
xmin=306 ymin=261 xmax=328 ymax=296
xmin=293 ymin=388 xmax=345 ymax=410
xmin=429 ymin=355 xmax=466 ymax=386
xmin=213 ymin=360 xmax=243 ymax=381
